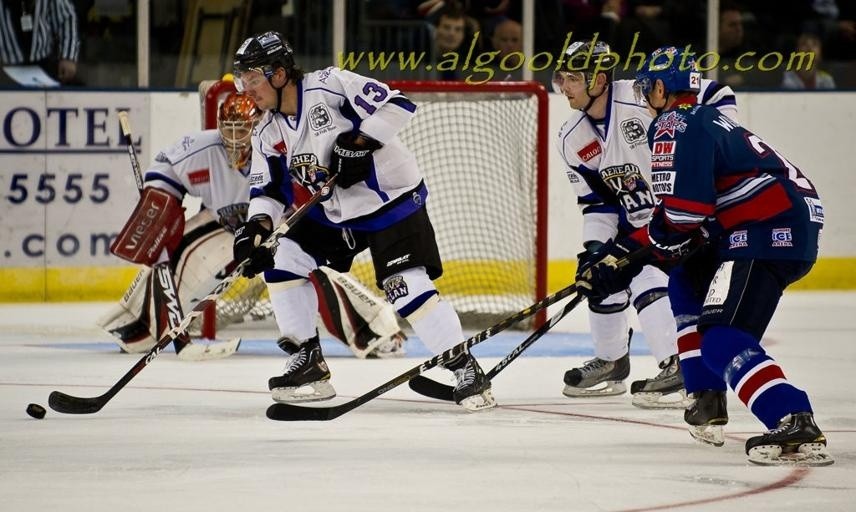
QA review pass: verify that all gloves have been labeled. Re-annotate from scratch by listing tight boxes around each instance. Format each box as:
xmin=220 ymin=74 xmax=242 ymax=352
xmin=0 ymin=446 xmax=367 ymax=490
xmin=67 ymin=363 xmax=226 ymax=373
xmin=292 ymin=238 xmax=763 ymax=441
xmin=232 ymin=221 xmax=275 ymax=279
xmin=576 ymin=240 xmax=644 ymax=298
xmin=329 ymin=132 xmax=373 ymax=189
xmin=110 ymin=185 xmax=186 ymax=267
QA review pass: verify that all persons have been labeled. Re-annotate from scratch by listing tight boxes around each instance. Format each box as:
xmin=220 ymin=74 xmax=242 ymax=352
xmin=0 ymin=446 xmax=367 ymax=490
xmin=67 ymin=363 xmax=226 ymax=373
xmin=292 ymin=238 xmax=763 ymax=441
xmin=230 ymin=29 xmax=498 ymax=412
xmin=717 ymin=7 xmax=747 ymax=92
xmin=783 ymin=35 xmax=836 ymax=91
xmin=1 ymin=1 xmax=81 ymax=92
xmin=630 ymin=45 xmax=835 ymax=468
xmin=471 ymin=20 xmax=525 ymax=81
xmin=550 ymin=40 xmax=738 ymax=413
xmin=93 ymin=92 xmax=408 ymax=356
xmin=400 ymin=5 xmax=471 ymax=81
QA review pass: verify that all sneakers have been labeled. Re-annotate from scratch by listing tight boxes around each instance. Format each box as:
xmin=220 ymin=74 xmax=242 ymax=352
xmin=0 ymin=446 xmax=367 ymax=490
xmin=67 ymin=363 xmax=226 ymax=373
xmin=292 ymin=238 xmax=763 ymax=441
xmin=631 ymin=354 xmax=689 ymax=395
xmin=268 ymin=326 xmax=332 ymax=391
xmin=683 ymin=390 xmax=728 ymax=426
xmin=563 ymin=351 xmax=631 ymax=388
xmin=440 ymin=349 xmax=492 ymax=405
xmin=746 ymin=413 xmax=827 ymax=456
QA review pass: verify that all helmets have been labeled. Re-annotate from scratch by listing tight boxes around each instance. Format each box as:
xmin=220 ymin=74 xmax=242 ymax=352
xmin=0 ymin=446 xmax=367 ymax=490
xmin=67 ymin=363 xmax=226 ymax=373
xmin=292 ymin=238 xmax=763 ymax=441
xmin=232 ymin=30 xmax=296 ymax=92
xmin=217 ymin=91 xmax=264 ymax=153
xmin=553 ymin=40 xmax=614 ymax=94
xmin=632 ymin=48 xmax=702 ymax=104
